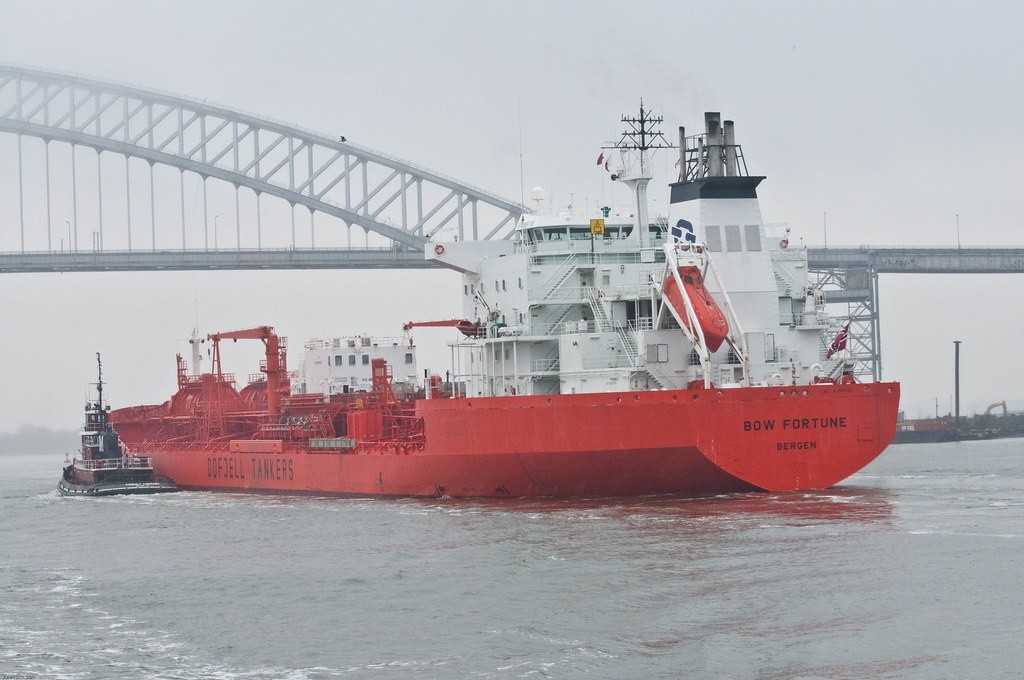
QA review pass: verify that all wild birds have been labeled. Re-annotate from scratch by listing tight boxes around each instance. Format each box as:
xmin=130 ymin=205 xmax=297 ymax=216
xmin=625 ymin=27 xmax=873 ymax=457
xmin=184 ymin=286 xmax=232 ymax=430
xmin=340 ymin=136 xmax=346 ymax=142
xmin=425 ymin=234 xmax=429 ymax=238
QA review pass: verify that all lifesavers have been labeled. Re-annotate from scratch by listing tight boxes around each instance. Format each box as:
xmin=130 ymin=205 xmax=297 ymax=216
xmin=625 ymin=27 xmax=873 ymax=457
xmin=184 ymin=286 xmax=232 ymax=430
xmin=780 ymin=240 xmax=788 ymax=249
xmin=435 ymin=245 xmax=445 ymax=254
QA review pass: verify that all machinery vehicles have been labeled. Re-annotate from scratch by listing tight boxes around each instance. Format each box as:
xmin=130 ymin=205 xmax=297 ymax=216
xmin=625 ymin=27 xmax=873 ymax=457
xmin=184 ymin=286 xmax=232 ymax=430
xmin=982 ymin=400 xmax=1009 ymax=419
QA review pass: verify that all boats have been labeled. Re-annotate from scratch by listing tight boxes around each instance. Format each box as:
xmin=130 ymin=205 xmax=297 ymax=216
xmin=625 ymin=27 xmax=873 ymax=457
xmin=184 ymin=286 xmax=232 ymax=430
xmin=57 ymin=350 xmax=181 ymax=495
xmin=107 ymin=100 xmax=903 ymax=496
xmin=891 ymin=415 xmax=962 ymax=444
xmin=662 ymin=266 xmax=728 ymax=354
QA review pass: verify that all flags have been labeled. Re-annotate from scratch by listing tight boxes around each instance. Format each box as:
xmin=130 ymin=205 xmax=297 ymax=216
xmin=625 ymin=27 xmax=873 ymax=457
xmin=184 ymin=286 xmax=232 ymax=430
xmin=605 ymin=155 xmax=612 ymax=172
xmin=596 ymin=151 xmax=604 ymax=166
xmin=826 ymin=324 xmax=849 ymax=359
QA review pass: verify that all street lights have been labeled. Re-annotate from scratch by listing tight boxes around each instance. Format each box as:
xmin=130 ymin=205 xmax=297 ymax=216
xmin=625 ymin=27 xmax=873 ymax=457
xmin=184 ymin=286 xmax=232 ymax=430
xmin=952 ymin=340 xmax=962 ymax=421
